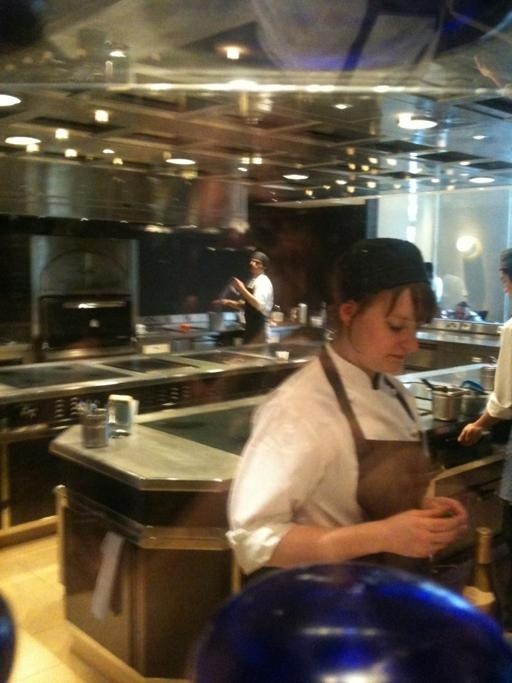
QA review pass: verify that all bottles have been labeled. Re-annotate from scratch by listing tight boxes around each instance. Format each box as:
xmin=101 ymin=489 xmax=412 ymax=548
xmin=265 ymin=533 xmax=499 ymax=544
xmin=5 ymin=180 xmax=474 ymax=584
xmin=319 ymin=299 xmax=327 ymax=319
xmin=459 ymin=527 xmax=501 ymax=621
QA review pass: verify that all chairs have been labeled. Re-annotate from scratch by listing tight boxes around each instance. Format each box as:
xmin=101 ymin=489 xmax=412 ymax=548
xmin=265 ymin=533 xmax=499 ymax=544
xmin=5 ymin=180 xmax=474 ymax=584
xmin=0 ymin=593 xmax=13 ymax=683
xmin=191 ymin=565 xmax=512 ymax=683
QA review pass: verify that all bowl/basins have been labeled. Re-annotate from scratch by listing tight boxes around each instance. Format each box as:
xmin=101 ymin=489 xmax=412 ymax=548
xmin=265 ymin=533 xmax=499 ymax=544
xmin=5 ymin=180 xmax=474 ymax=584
xmin=460 ymin=390 xmax=491 ymax=416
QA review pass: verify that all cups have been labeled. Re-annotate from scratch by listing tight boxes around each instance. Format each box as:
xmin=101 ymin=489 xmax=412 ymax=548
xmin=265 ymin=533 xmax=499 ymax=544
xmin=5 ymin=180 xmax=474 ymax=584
xmin=431 ymin=387 xmax=461 ymax=422
xmin=107 ymin=400 xmax=139 ymax=434
xmin=479 ymin=365 xmax=496 ymax=391
xmin=82 ymin=408 xmax=110 ymax=447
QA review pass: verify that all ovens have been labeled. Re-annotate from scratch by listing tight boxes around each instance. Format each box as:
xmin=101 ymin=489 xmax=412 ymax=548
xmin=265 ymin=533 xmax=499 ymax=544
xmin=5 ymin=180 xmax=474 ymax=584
xmin=35 ymin=293 xmax=142 ymax=360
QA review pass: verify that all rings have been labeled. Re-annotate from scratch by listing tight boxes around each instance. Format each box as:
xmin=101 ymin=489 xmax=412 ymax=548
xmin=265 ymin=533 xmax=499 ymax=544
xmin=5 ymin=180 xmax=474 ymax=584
xmin=428 ymin=553 xmax=435 ymax=560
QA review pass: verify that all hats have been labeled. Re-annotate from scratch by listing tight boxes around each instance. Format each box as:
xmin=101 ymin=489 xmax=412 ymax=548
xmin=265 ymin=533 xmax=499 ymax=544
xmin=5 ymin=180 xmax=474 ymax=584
xmin=499 ymin=248 xmax=512 ymax=269
xmin=249 ymin=250 xmax=269 ymax=266
xmin=336 ymin=238 xmax=426 ymax=287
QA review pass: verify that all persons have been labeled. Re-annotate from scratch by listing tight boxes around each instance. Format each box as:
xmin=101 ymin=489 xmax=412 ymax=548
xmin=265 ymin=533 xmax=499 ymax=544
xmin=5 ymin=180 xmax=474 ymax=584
xmin=459 ymin=248 xmax=512 ymax=554
xmin=183 ymin=294 xmax=204 ymax=313
xmin=228 ymin=238 xmax=469 ymax=580
xmin=209 ymin=250 xmax=274 ymax=342
xmin=423 ymin=261 xmax=442 ymax=303
xmin=444 ymin=302 xmax=481 ymax=323
